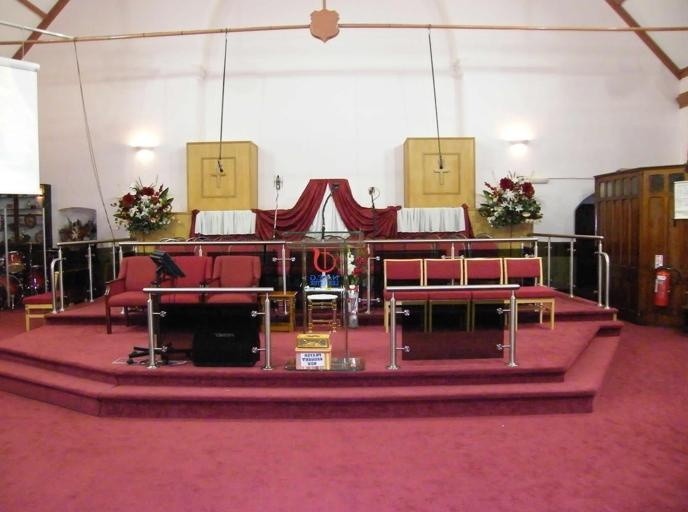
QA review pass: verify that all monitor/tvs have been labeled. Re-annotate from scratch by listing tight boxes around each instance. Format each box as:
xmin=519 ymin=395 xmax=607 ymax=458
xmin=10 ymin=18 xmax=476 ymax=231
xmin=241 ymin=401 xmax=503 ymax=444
xmin=150 ymin=250 xmax=185 ymax=279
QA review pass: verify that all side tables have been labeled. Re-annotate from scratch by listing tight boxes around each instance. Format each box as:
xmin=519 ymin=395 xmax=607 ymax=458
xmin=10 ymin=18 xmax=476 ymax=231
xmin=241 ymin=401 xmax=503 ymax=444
xmin=259 ymin=291 xmax=298 ymax=333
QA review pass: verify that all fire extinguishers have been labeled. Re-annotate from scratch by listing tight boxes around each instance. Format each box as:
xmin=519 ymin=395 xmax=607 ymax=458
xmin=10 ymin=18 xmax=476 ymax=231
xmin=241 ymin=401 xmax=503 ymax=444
xmin=653 ymin=265 xmax=673 ymax=309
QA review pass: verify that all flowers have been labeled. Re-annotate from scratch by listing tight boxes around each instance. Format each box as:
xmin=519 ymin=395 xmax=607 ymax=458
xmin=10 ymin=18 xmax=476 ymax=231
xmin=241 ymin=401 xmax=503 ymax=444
xmin=109 ymin=174 xmax=178 ymax=234
xmin=59 ymin=216 xmax=97 ymax=249
xmin=477 ymin=168 xmax=545 ymax=228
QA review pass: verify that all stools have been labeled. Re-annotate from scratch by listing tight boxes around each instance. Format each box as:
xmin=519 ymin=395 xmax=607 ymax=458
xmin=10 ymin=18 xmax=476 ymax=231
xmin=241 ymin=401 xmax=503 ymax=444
xmin=307 ymin=294 xmax=338 ymax=335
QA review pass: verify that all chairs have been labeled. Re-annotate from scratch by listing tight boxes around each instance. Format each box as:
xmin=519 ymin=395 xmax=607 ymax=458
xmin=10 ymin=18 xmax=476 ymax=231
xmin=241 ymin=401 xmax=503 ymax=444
xmin=382 ymin=254 xmax=556 ymax=333
xmin=23 ymin=257 xmax=66 ymax=333
xmin=103 ymin=254 xmax=263 ymax=334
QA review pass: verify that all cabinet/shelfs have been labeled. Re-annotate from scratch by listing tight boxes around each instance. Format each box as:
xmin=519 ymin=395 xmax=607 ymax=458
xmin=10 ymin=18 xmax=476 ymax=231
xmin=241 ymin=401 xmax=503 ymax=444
xmin=0 ymin=184 xmax=52 ymax=311
xmin=594 ymin=163 xmax=688 ymax=326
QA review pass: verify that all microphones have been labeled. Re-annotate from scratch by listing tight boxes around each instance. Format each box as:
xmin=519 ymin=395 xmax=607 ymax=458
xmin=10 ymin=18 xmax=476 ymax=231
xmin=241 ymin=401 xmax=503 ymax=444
xmin=368 ymin=187 xmax=375 ymax=195
xmin=321 ymin=183 xmax=340 ymax=243
xmin=276 ymin=175 xmax=280 ymax=190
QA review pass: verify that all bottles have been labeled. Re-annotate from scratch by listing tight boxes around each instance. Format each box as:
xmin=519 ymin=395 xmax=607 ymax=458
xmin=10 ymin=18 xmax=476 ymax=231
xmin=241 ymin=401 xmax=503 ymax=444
xmin=320 ymin=272 xmax=327 ymax=288
